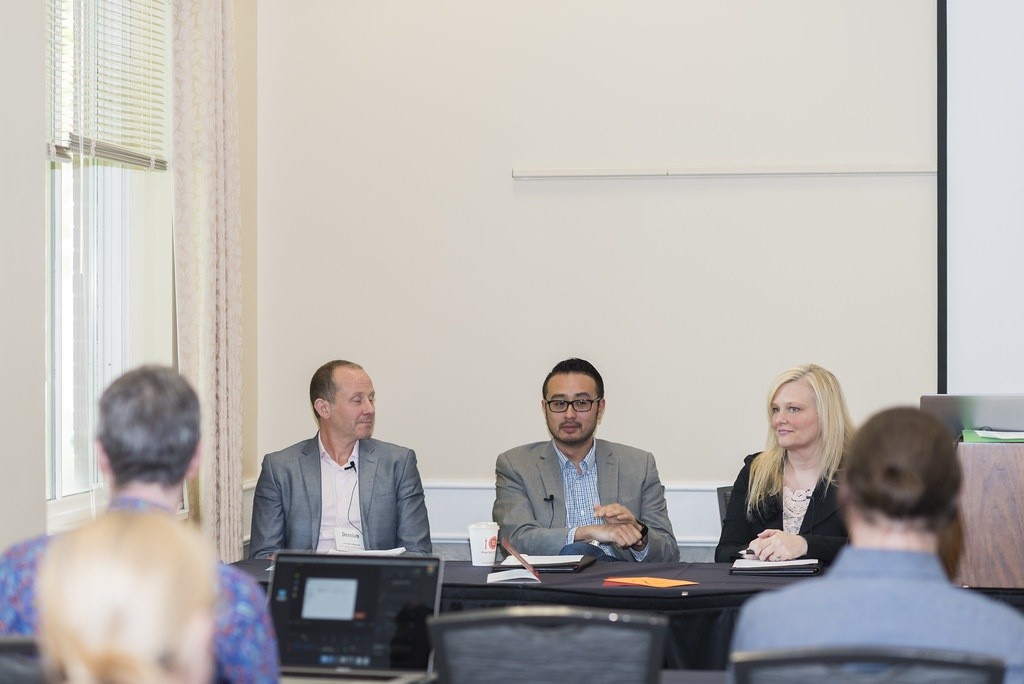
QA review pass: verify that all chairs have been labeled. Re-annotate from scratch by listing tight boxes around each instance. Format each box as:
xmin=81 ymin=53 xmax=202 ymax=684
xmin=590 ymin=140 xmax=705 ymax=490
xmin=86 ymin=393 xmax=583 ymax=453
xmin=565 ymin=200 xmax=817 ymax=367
xmin=430 ymin=602 xmax=1006 ymax=683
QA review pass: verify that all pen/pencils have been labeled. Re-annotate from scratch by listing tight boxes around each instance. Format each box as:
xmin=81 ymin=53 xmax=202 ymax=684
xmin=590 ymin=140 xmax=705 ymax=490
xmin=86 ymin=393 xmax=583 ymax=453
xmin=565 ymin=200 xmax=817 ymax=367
xmin=737 ymin=549 xmax=755 ymax=556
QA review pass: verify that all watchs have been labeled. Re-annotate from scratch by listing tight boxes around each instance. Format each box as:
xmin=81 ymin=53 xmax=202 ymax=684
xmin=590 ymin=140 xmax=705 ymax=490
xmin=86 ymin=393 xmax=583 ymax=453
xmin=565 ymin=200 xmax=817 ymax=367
xmin=636 ymin=519 xmax=648 ymax=537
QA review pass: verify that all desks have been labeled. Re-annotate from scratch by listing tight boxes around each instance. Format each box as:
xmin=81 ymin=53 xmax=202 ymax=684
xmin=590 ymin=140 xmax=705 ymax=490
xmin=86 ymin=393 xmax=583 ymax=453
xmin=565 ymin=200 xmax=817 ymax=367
xmin=233 ymin=550 xmax=829 ymax=596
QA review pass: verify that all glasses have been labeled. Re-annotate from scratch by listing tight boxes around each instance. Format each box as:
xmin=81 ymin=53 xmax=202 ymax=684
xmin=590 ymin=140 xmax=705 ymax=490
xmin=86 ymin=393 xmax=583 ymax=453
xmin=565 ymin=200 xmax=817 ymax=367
xmin=546 ymin=396 xmax=601 ymax=412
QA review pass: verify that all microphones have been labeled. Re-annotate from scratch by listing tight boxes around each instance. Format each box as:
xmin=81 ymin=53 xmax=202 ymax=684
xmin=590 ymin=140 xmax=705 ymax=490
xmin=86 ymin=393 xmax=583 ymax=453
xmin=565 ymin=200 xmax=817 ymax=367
xmin=550 ymin=495 xmax=554 ymax=507
xmin=806 ymin=496 xmax=814 ymax=501
xmin=350 ymin=461 xmax=357 ymax=472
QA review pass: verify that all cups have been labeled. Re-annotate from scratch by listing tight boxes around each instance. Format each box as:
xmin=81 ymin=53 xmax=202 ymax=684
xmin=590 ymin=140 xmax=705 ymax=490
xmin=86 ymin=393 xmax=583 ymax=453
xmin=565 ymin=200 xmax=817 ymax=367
xmin=468 ymin=521 xmax=498 ymax=566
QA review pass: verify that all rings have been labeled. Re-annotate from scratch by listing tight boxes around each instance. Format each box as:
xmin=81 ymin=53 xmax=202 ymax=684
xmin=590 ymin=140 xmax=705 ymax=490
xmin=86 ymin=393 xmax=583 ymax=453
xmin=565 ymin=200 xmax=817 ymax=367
xmin=772 ymin=553 xmax=780 ymax=562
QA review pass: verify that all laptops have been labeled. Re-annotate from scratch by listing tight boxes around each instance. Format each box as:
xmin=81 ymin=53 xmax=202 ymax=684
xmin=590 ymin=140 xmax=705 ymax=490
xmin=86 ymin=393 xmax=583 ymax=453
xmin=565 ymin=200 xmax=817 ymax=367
xmin=267 ymin=549 xmax=446 ymax=684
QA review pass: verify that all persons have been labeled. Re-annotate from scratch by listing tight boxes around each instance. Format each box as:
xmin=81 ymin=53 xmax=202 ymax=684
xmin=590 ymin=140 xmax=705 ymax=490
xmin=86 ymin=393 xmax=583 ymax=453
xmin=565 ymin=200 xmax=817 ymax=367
xmin=714 ymin=366 xmax=1024 ymax=684
xmin=0 ymin=359 xmax=434 ymax=684
xmin=492 ymin=359 xmax=681 ymax=562
